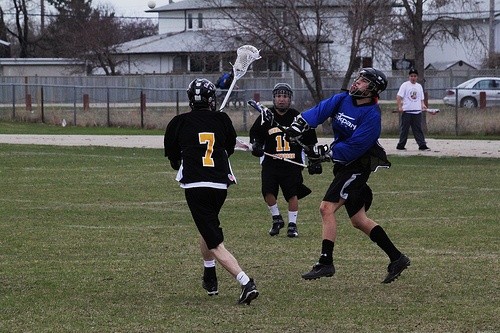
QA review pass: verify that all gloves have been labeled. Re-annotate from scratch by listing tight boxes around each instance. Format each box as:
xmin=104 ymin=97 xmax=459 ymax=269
xmin=251 ymin=139 xmax=264 ymax=157
xmin=282 ymin=113 xmax=311 ymax=144
xmin=303 ymin=144 xmax=331 ymax=163
xmin=308 ymin=163 xmax=322 ymax=175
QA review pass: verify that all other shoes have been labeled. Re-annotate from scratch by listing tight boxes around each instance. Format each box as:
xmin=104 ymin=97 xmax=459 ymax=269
xmin=396 ymin=146 xmax=407 ymax=152
xmin=418 ymin=145 xmax=431 ymax=151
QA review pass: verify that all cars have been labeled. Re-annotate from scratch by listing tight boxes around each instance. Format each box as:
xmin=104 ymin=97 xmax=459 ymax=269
xmin=443 ymin=77 xmax=500 ymax=108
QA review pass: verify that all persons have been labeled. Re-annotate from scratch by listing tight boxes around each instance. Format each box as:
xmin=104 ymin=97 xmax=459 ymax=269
xmin=284 ymin=68 xmax=410 ymax=285
xmin=395 ymin=69 xmax=431 ymax=150
xmin=249 ymin=83 xmax=323 ymax=236
xmin=164 ymin=68 xmax=258 ymax=306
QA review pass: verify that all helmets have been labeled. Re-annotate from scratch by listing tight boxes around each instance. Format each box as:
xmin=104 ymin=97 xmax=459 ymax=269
xmin=272 ymin=83 xmax=293 ymax=95
xmin=186 ymin=78 xmax=216 ymax=108
xmin=359 ymin=68 xmax=388 ymax=91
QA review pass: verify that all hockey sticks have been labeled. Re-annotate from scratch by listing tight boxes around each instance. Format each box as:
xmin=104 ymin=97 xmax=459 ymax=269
xmin=392 ymin=107 xmax=440 ymax=113
xmin=234 ymin=138 xmax=307 ymax=170
xmin=247 ymin=97 xmax=312 ymax=149
xmin=220 ymin=44 xmax=261 ymax=113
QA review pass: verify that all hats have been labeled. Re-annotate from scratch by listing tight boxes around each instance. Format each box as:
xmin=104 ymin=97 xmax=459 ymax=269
xmin=409 ymin=68 xmax=419 ymax=76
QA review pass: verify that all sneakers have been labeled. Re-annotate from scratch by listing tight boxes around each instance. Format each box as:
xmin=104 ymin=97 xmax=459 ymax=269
xmin=301 ymin=263 xmax=335 ymax=280
xmin=201 ymin=276 xmax=218 ymax=296
xmin=381 ymin=254 xmax=411 ymax=284
xmin=269 ymin=215 xmax=285 ymax=236
xmin=238 ymin=278 xmax=259 ymax=304
xmin=287 ymin=222 xmax=299 ymax=237
